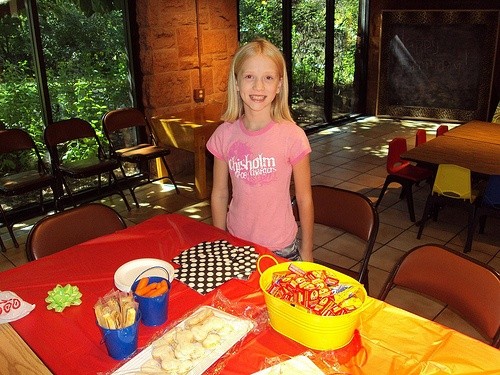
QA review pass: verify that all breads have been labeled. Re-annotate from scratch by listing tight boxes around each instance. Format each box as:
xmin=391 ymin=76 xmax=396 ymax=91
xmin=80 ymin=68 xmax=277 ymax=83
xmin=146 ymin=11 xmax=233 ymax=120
xmin=135 ymin=309 xmax=235 ymax=375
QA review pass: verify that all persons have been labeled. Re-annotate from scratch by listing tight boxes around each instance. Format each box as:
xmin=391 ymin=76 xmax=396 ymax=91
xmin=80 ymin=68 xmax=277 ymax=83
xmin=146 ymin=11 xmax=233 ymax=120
xmin=206 ymin=39 xmax=314 ymax=260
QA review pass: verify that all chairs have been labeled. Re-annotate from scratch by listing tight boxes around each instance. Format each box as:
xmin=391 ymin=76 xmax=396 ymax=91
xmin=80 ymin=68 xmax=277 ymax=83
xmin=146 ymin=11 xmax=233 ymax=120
xmin=25 ymin=201 xmax=128 ymax=263
xmin=102 ymin=107 xmax=180 ymax=209
xmin=288 ymin=184 xmax=381 ymax=295
xmin=374 ymin=124 xmax=500 ymax=256
xmin=44 ymin=118 xmax=131 ymax=215
xmin=377 ymin=242 xmax=500 ymax=351
xmin=0 ymin=128 xmax=62 ymax=248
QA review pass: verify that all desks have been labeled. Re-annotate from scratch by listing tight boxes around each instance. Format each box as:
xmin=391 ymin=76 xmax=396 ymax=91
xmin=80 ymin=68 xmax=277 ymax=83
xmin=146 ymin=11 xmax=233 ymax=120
xmin=399 ymin=119 xmax=500 ymax=237
xmin=0 ymin=212 xmax=500 ymax=375
xmin=148 ymin=105 xmax=228 ymax=201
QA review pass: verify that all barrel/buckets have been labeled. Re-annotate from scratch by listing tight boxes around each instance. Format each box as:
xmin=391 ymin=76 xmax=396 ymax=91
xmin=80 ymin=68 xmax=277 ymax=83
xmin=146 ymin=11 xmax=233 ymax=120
xmin=132 ymin=266 xmax=171 ymax=326
xmin=257 ymin=254 xmax=373 ymax=351
xmin=96 ymin=310 xmax=142 ymax=360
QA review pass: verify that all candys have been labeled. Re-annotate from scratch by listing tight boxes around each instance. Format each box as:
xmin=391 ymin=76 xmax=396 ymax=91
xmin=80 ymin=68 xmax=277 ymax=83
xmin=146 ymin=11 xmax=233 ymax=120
xmin=266 ymin=263 xmax=363 ymax=316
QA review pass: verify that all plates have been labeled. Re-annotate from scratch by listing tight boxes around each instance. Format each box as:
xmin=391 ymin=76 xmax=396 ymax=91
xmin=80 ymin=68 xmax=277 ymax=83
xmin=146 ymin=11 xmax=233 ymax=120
xmin=114 ymin=258 xmax=175 ymax=293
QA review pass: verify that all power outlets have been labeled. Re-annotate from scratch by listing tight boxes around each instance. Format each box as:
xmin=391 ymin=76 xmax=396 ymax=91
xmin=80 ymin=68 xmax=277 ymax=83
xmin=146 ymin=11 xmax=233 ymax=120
xmin=193 ymin=87 xmax=205 ymax=102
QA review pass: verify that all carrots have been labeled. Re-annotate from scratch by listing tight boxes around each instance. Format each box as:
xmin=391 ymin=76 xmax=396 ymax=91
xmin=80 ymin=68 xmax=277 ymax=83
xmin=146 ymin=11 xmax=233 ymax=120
xmin=135 ymin=278 xmax=168 ymax=298
